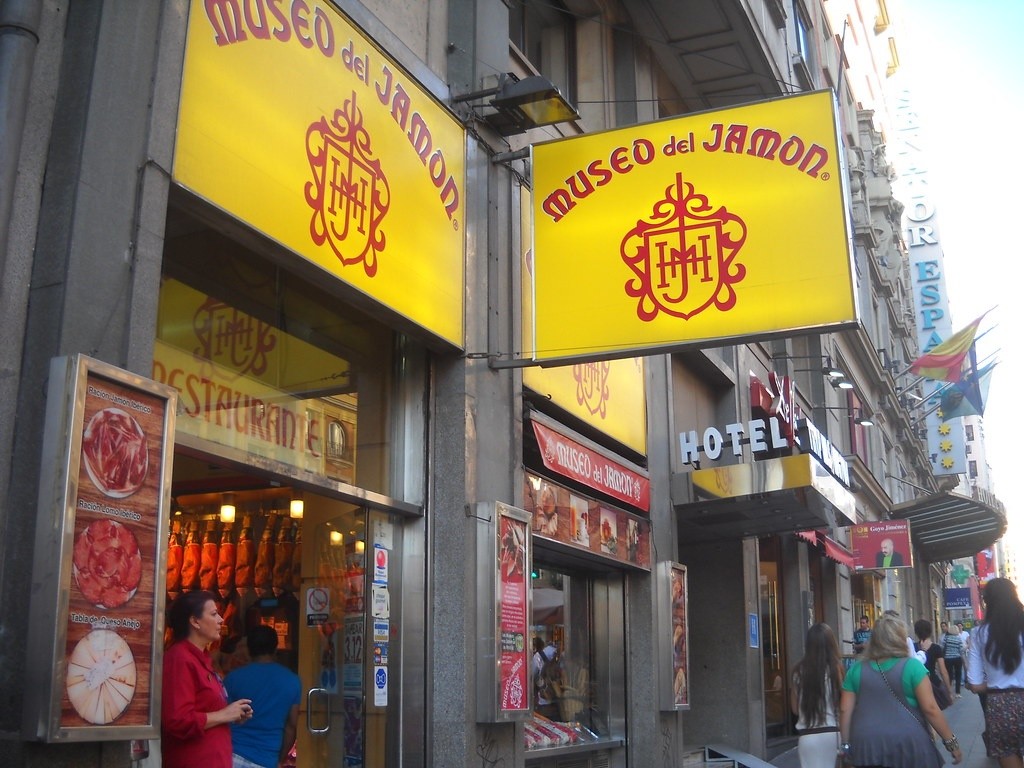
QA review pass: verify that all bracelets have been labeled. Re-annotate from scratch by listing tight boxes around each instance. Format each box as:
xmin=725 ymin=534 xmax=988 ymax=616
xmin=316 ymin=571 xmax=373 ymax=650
xmin=841 ymin=744 xmax=851 ymax=751
xmin=943 ymin=736 xmax=959 ymax=752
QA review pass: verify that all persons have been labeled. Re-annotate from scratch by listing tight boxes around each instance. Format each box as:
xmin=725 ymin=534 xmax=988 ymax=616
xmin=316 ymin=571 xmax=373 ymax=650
xmin=223 ymin=625 xmax=301 ymax=768
xmin=532 ymin=637 xmax=562 ymax=722
xmin=790 ymin=578 xmax=1024 ymax=768
xmin=160 ymin=591 xmax=253 ymax=768
xmin=876 ymin=539 xmax=903 ymax=567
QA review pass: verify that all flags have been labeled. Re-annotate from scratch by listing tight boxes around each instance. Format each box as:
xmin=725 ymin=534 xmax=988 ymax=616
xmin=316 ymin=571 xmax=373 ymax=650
xmin=909 ymin=316 xmax=992 ymax=422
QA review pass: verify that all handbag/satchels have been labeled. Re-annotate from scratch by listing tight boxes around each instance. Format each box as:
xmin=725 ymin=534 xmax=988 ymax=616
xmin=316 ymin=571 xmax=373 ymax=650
xmin=933 ymin=682 xmax=953 ymax=710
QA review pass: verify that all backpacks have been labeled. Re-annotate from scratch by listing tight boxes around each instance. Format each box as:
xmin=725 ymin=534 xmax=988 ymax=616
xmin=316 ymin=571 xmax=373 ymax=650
xmin=537 ymin=650 xmax=563 ymax=700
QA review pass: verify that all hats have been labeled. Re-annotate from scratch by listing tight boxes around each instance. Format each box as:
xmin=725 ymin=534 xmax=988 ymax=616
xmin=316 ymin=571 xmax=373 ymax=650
xmin=947 ymin=625 xmax=959 ymax=635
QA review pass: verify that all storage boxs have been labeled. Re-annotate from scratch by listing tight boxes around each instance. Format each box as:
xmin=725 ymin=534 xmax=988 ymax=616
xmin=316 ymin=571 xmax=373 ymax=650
xmin=262 ymin=614 xmax=294 ymax=651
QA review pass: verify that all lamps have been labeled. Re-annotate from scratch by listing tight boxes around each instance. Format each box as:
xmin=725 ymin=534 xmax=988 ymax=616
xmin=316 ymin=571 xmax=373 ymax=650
xmin=908 ymin=398 xmax=916 ymax=410
xmin=171 ymin=499 xmax=184 ymax=516
xmin=929 ymin=454 xmax=937 ymax=463
xmin=772 ymin=355 xmax=875 ymax=427
xmin=290 ymin=486 xmax=304 ymax=519
xmin=885 ymin=360 xmax=901 ymax=374
xmin=457 ymin=75 xmax=583 ymax=133
xmin=220 ymin=493 xmax=236 ymax=523
xmin=918 ymin=429 xmax=928 ymax=442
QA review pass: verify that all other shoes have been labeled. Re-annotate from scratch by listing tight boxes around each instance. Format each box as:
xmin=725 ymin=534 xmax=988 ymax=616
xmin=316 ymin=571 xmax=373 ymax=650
xmin=955 ymin=694 xmax=962 ymax=698
